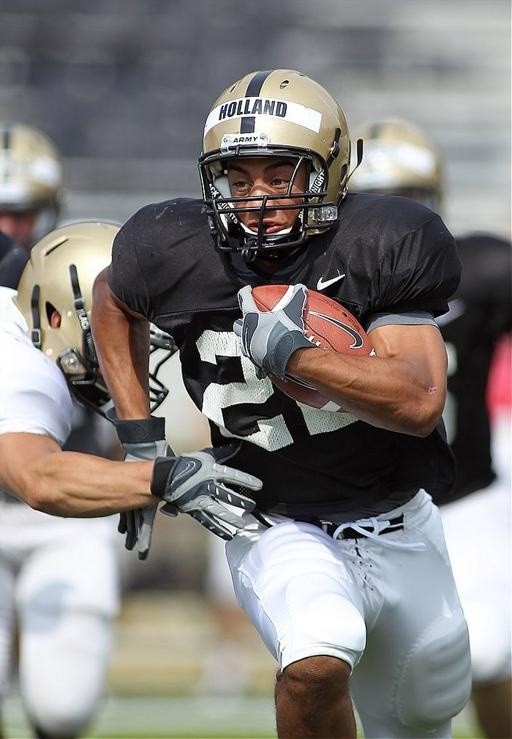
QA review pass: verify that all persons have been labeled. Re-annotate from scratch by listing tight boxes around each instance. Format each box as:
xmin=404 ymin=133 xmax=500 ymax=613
xmin=0 ymin=123 xmax=263 ymax=739
xmin=89 ymin=68 xmax=475 ymax=739
xmin=342 ymin=125 xmax=511 ymax=739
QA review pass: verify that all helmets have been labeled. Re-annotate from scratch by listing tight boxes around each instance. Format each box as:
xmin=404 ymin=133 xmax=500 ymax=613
xmin=349 ymin=121 xmax=442 ymax=199
xmin=12 ymin=217 xmax=180 ymax=430
xmin=198 ymin=69 xmax=352 ymax=237
xmin=0 ymin=124 xmax=66 ymax=215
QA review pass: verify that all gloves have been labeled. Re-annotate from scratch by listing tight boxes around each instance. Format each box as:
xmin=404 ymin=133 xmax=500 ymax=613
xmin=103 ymin=404 xmax=178 ymax=562
xmin=148 ymin=438 xmax=264 ymax=542
xmin=232 ymin=281 xmax=321 ymax=385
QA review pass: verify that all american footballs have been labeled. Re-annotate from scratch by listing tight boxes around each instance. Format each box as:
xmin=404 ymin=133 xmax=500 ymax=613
xmin=251 ymin=285 xmax=372 ymax=413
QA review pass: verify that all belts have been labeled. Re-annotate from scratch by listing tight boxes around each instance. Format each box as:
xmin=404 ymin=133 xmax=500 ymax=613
xmin=300 ymin=516 xmax=408 ymax=538
